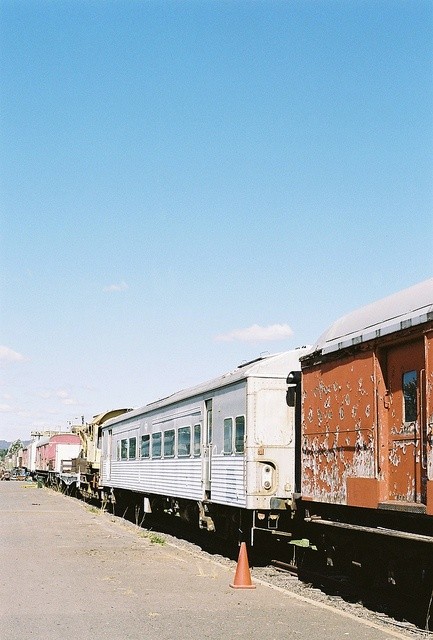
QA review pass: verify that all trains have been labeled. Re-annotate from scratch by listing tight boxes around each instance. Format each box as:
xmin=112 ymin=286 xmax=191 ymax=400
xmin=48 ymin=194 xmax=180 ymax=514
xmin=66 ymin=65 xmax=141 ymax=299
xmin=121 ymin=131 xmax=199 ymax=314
xmin=3 ymin=273 xmax=432 ymax=630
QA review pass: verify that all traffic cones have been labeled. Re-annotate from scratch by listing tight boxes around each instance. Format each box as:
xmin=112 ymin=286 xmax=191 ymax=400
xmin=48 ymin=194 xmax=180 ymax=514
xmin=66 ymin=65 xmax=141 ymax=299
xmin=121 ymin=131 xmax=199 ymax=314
xmin=228 ymin=542 xmax=255 ymax=589
